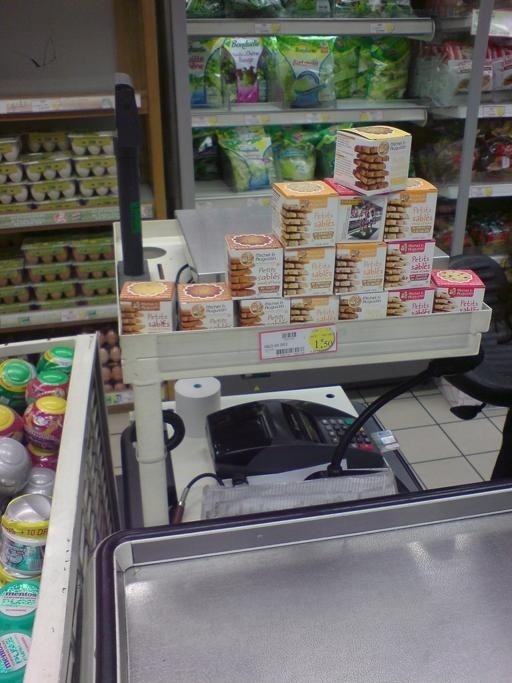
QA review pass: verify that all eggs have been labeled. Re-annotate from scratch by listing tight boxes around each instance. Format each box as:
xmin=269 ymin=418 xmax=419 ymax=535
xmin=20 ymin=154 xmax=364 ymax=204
xmin=1 ymin=140 xmax=118 ymax=304
xmin=92 ymin=328 xmax=135 ymax=394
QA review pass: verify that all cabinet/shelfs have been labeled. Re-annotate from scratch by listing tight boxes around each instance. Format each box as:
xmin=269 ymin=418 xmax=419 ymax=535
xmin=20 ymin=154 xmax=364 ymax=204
xmin=115 ymin=0 xmax=512 ymax=269
xmin=0 ymin=92 xmax=164 ymax=409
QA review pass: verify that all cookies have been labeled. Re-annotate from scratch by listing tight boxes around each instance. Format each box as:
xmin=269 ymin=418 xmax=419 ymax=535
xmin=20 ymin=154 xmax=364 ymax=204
xmin=120 ymin=145 xmax=457 ymax=334
